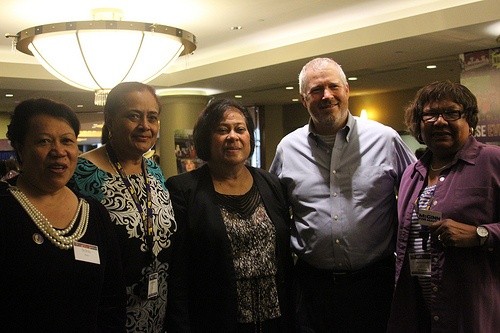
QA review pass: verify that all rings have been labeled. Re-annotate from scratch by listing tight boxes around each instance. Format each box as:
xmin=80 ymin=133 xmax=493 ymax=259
xmin=438 ymin=235 xmax=441 ymax=240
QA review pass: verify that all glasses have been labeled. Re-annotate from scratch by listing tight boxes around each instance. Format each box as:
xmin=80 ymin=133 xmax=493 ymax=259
xmin=419 ymin=110 xmax=465 ymax=123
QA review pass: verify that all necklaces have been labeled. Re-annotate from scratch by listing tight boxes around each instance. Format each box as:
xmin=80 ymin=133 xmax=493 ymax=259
xmin=430 ymin=159 xmax=450 ymax=171
xmin=7 ymin=185 xmax=90 ymax=250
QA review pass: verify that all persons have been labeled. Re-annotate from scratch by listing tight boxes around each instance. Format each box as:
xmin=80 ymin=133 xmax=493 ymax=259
xmin=185 ymin=160 xmax=196 ymax=172
xmin=386 ymin=80 xmax=500 ymax=333
xmin=0 ymin=98 xmax=127 ymax=333
xmin=64 ymin=82 xmax=177 ymax=332
xmin=269 ymin=58 xmax=418 ymax=333
xmin=164 ymin=99 xmax=289 ymax=333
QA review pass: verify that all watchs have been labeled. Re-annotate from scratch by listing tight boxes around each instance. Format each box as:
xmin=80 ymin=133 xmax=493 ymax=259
xmin=475 ymin=226 xmax=489 ymax=245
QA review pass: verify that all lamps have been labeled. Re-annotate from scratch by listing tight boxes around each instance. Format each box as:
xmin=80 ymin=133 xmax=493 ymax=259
xmin=5 ymin=0 xmax=198 ymax=107
